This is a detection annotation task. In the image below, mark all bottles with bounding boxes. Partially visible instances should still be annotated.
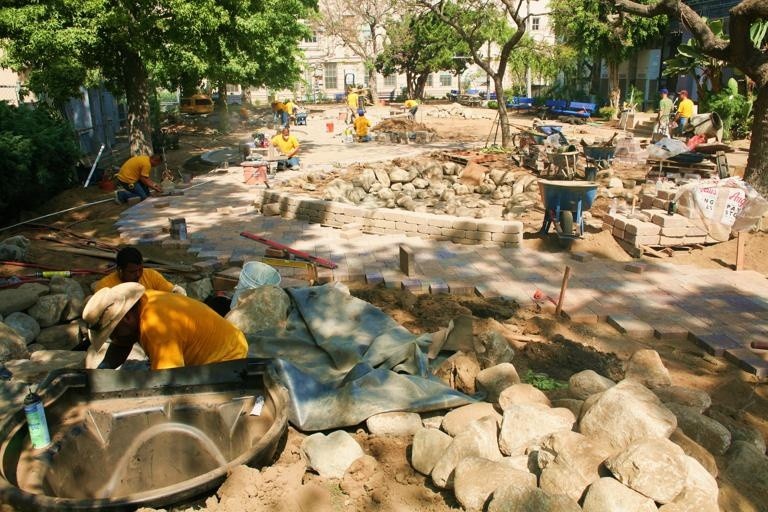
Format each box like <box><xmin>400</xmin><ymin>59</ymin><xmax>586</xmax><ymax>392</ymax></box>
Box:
<box><xmin>23</xmin><ymin>388</ymin><xmax>52</xmax><ymax>451</ymax></box>
<box><xmin>35</xmin><ymin>271</ymin><xmax>71</xmax><ymax>278</ymax></box>
<box><xmin>178</xmin><ymin>222</ymin><xmax>186</xmax><ymax>241</ymax></box>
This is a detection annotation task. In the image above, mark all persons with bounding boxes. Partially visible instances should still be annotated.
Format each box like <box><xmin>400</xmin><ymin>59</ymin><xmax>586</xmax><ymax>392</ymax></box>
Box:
<box><xmin>80</xmin><ymin>247</ymin><xmax>187</xmax><ymax>336</ymax></box>
<box><xmin>405</xmin><ymin>99</ymin><xmax>419</xmax><ymax>116</ymax></box>
<box><xmin>696</xmin><ymin>72</ymin><xmax>712</xmax><ymax>91</ymax></box>
<box><xmin>674</xmin><ymin>90</ymin><xmax>694</xmax><ymax>133</ymax></box>
<box><xmin>272</xmin><ymin>97</ymin><xmax>306</xmax><ymax>129</ymax></box>
<box><xmin>346</xmin><ymin>84</ymin><xmax>373</xmax><ymax>142</ymax></box>
<box><xmin>115</xmin><ymin>154</ymin><xmax>163</xmax><ymax>206</ymax></box>
<box><xmin>271</xmin><ymin>128</ymin><xmax>300</xmax><ymax>171</ymax></box>
<box><xmin>82</xmin><ymin>282</ymin><xmax>248</xmax><ymax>371</ymax></box>
<box><xmin>652</xmin><ymin>88</ymin><xmax>674</xmax><ymax>142</ymax></box>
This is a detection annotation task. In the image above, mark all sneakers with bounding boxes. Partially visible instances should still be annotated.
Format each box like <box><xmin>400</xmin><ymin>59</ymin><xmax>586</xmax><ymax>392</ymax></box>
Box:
<box><xmin>114</xmin><ymin>191</ymin><xmax>121</xmax><ymax>205</ymax></box>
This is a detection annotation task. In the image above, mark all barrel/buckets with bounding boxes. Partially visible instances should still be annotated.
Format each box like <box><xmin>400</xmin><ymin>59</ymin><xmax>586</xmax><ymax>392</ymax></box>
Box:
<box><xmin>339</xmin><ymin>112</ymin><xmax>348</xmax><ymax>122</ymax></box>
<box><xmin>326</xmin><ymin>121</ymin><xmax>333</xmax><ymax>132</ymax></box>
<box><xmin>230</xmin><ymin>258</ymin><xmax>280</xmax><ymax>310</ymax></box>
<box><xmin>240</xmin><ymin>161</ymin><xmax>268</xmax><ymax>184</ymax></box>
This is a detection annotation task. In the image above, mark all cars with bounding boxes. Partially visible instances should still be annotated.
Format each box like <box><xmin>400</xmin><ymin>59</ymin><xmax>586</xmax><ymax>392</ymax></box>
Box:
<box><xmin>179</xmin><ymin>92</ymin><xmax>214</xmax><ymax>114</ymax></box>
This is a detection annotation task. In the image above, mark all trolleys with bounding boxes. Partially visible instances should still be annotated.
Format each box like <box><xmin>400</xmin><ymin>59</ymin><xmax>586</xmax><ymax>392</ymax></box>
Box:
<box><xmin>535</xmin><ymin>139</ymin><xmax>616</xmax><ymax>248</ymax></box>
<box><xmin>295</xmin><ymin>113</ymin><xmax>308</xmax><ymax>125</ymax></box>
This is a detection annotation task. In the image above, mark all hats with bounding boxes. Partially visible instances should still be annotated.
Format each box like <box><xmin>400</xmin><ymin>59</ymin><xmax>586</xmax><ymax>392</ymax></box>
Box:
<box><xmin>677</xmin><ymin>90</ymin><xmax>688</xmax><ymax>95</ymax></box>
<box><xmin>658</xmin><ymin>88</ymin><xmax>668</xmax><ymax>94</ymax></box>
<box><xmin>82</xmin><ymin>282</ymin><xmax>145</xmax><ymax>353</ymax></box>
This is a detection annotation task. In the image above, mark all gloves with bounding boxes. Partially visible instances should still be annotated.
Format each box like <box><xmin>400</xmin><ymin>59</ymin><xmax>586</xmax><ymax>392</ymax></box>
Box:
<box><xmin>172</xmin><ymin>284</ymin><xmax>187</xmax><ymax>296</ymax></box>
